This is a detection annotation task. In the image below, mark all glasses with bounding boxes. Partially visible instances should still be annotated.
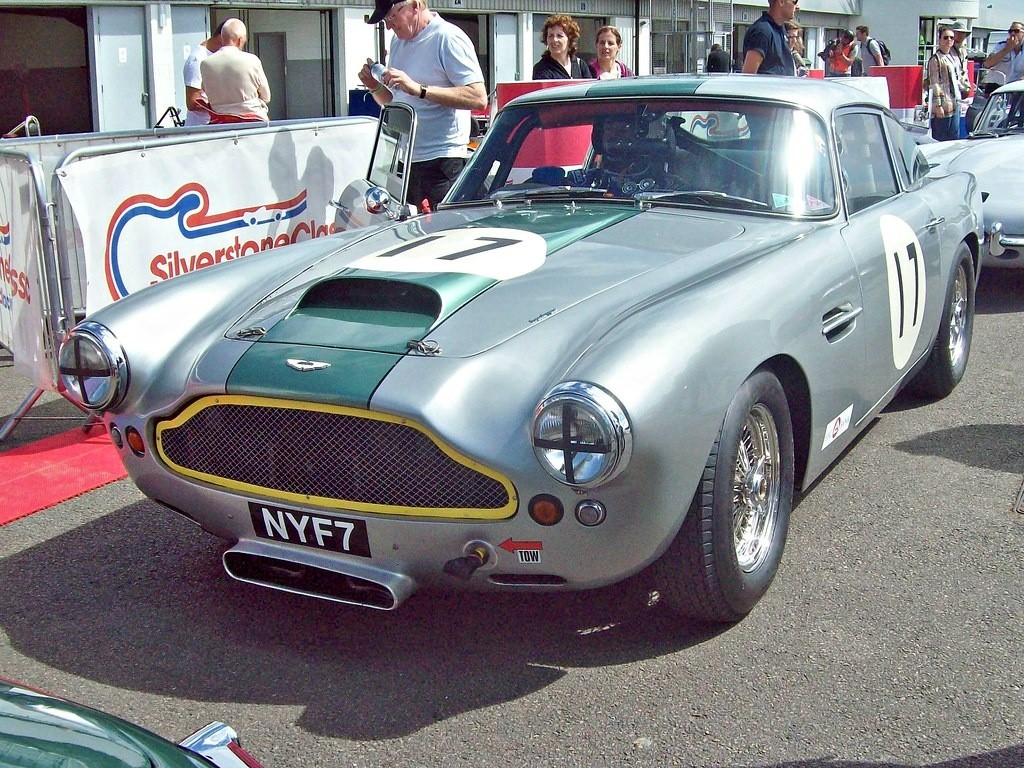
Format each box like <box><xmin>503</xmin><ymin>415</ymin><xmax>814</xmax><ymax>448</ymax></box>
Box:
<box><xmin>1008</xmin><ymin>28</ymin><xmax>1023</xmax><ymax>34</ymax></box>
<box><xmin>942</xmin><ymin>36</ymin><xmax>954</xmax><ymax>40</ymax></box>
<box><xmin>789</xmin><ymin>0</ymin><xmax>798</xmax><ymax>5</ymax></box>
<box><xmin>383</xmin><ymin>5</ymin><xmax>414</xmax><ymax>23</ymax></box>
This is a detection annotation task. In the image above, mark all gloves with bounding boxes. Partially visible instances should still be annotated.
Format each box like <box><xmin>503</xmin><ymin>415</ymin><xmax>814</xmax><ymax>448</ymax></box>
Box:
<box><xmin>532</xmin><ymin>166</ymin><xmax>565</xmax><ymax>186</ymax></box>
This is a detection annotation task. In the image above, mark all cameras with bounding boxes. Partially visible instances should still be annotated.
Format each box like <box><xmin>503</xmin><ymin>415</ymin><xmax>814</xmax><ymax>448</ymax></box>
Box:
<box><xmin>818</xmin><ymin>43</ymin><xmax>837</xmax><ymax>61</ymax></box>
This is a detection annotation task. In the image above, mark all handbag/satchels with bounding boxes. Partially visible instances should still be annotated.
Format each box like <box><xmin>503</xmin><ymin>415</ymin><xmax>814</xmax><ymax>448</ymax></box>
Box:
<box><xmin>931</xmin><ymin>93</ymin><xmax>953</xmax><ymax>113</ymax></box>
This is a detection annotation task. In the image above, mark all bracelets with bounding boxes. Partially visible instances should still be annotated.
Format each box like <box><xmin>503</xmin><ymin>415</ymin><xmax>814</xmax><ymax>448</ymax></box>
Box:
<box><xmin>936</xmin><ymin>104</ymin><xmax>942</xmax><ymax>107</ymax></box>
<box><xmin>367</xmin><ymin>84</ymin><xmax>384</xmax><ymax>95</ymax></box>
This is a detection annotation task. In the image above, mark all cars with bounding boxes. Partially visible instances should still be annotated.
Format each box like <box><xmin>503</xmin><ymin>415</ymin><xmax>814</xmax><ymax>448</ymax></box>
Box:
<box><xmin>1</xmin><ymin>676</ymin><xmax>264</xmax><ymax>768</ymax></box>
<box><xmin>57</xmin><ymin>74</ymin><xmax>984</xmax><ymax>618</ymax></box>
<box><xmin>917</xmin><ymin>79</ymin><xmax>1024</xmax><ymax>255</ymax></box>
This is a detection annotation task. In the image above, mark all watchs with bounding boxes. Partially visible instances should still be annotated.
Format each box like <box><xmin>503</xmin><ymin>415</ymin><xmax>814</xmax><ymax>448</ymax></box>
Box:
<box><xmin>419</xmin><ymin>82</ymin><xmax>429</xmax><ymax>99</ymax></box>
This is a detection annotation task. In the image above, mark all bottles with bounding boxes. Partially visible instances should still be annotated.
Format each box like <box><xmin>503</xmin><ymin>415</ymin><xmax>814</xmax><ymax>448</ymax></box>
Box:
<box><xmin>370</xmin><ymin>62</ymin><xmax>404</xmax><ymax>96</ymax></box>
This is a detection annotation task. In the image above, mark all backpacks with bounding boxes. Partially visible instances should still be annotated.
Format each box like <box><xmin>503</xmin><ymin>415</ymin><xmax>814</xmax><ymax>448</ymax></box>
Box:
<box><xmin>860</xmin><ymin>38</ymin><xmax>891</xmax><ymax>66</ymax></box>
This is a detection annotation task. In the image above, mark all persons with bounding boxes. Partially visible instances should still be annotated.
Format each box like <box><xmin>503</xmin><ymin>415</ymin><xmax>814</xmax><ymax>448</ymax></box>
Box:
<box><xmin>985</xmin><ymin>22</ymin><xmax>1024</xmax><ymax>85</ymax></box>
<box><xmin>588</xmin><ymin>26</ymin><xmax>636</xmax><ymax>79</ymax></box>
<box><xmin>856</xmin><ymin>25</ymin><xmax>885</xmax><ymax>77</ymax></box>
<box><xmin>358</xmin><ymin>0</ymin><xmax>489</xmax><ymax>210</ymax></box>
<box><xmin>926</xmin><ymin>21</ymin><xmax>988</xmax><ymax>141</ymax></box>
<box><xmin>740</xmin><ymin>0</ymin><xmax>810</xmax><ymax>77</ymax></box>
<box><xmin>200</xmin><ymin>18</ymin><xmax>271</xmax><ymax>124</ymax></box>
<box><xmin>566</xmin><ymin>113</ymin><xmax>684</xmax><ymax>191</ymax></box>
<box><xmin>817</xmin><ymin>29</ymin><xmax>859</xmax><ymax>77</ymax></box>
<box><xmin>703</xmin><ymin>44</ymin><xmax>732</xmax><ymax>75</ymax></box>
<box><xmin>532</xmin><ymin>14</ymin><xmax>593</xmax><ymax>80</ymax></box>
<box><xmin>183</xmin><ymin>17</ymin><xmax>227</xmax><ymax>127</ymax></box>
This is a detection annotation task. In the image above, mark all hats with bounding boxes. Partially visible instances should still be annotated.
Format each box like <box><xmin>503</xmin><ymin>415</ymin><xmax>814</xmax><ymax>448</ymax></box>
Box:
<box><xmin>951</xmin><ymin>21</ymin><xmax>971</xmax><ymax>37</ymax></box>
<box><xmin>366</xmin><ymin>0</ymin><xmax>409</xmax><ymax>24</ymax></box>
